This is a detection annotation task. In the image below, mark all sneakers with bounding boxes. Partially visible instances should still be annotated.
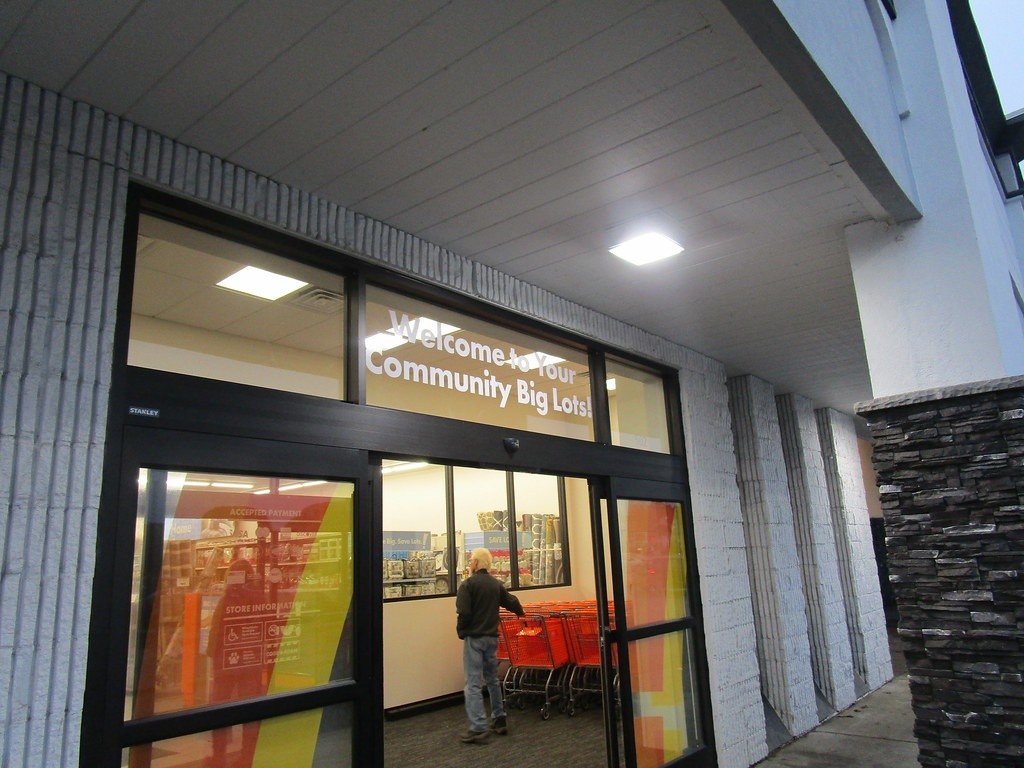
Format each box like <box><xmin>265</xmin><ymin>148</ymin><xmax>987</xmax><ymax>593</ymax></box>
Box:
<box><xmin>489</xmin><ymin>716</ymin><xmax>508</xmax><ymax>734</ymax></box>
<box><xmin>460</xmin><ymin>725</ymin><xmax>489</xmax><ymax>742</ymax></box>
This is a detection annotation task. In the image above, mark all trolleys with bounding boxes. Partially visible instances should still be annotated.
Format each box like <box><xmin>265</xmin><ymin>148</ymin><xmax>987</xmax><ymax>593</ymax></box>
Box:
<box><xmin>496</xmin><ymin>599</ymin><xmax>618</xmax><ymax>721</ymax></box>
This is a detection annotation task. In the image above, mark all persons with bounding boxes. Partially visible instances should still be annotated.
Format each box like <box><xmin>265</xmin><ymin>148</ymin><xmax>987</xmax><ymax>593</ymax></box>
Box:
<box><xmin>456</xmin><ymin>548</ymin><xmax>526</xmax><ymax>740</ymax></box>
<box><xmin>205</xmin><ymin>559</ymin><xmax>268</xmax><ymax>768</ymax></box>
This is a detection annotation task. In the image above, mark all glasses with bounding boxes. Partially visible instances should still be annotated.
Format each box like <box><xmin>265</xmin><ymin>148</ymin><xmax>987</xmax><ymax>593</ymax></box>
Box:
<box><xmin>468</xmin><ymin>559</ymin><xmax>476</xmax><ymax>564</ymax></box>
